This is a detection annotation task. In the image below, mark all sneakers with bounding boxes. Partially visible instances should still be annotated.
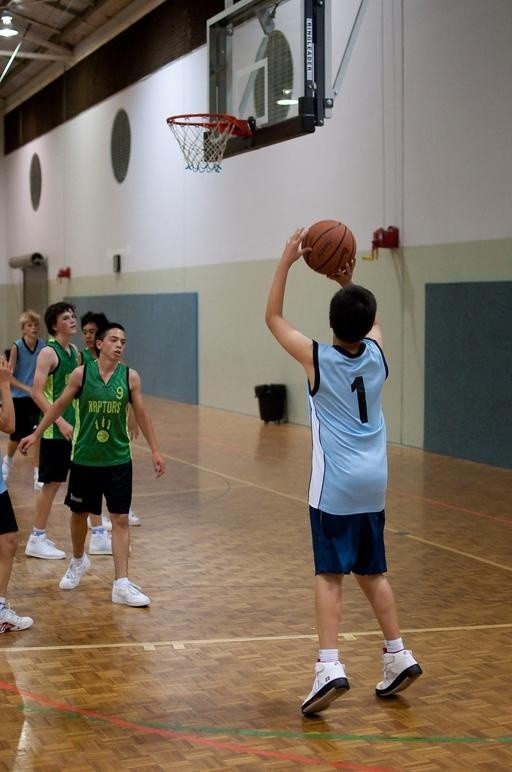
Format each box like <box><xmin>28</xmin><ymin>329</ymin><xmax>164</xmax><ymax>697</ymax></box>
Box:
<box><xmin>0</xmin><ymin>608</ymin><xmax>33</xmax><ymax>633</ymax></box>
<box><xmin>111</xmin><ymin>578</ymin><xmax>151</xmax><ymax>607</ymax></box>
<box><xmin>89</xmin><ymin>531</ymin><xmax>131</xmax><ymax>555</ymax></box>
<box><xmin>59</xmin><ymin>554</ymin><xmax>92</xmax><ymax>590</ymax></box>
<box><xmin>301</xmin><ymin>661</ymin><xmax>350</xmax><ymax>716</ymax></box>
<box><xmin>87</xmin><ymin>509</ymin><xmax>140</xmax><ymax>530</ymax></box>
<box><xmin>25</xmin><ymin>531</ymin><xmax>64</xmax><ymax>560</ymax></box>
<box><xmin>376</xmin><ymin>650</ymin><xmax>423</xmax><ymax>698</ymax></box>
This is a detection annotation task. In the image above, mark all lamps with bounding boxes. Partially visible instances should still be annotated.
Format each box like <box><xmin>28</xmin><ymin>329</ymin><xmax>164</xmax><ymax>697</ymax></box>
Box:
<box><xmin>0</xmin><ymin>8</ymin><xmax>19</xmax><ymax>37</ymax></box>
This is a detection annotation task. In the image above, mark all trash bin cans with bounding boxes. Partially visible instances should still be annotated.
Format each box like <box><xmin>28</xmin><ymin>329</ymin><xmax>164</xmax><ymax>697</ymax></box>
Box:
<box><xmin>255</xmin><ymin>384</ymin><xmax>286</xmax><ymax>424</ymax></box>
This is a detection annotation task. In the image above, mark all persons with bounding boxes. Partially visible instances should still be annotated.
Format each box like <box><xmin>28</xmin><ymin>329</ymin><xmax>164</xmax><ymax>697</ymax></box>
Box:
<box><xmin>265</xmin><ymin>225</ymin><xmax>422</xmax><ymax>715</ymax></box>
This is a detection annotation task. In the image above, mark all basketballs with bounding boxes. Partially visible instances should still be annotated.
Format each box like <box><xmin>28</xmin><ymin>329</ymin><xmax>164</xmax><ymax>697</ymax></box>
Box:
<box><xmin>301</xmin><ymin>220</ymin><xmax>356</xmax><ymax>275</ymax></box>
